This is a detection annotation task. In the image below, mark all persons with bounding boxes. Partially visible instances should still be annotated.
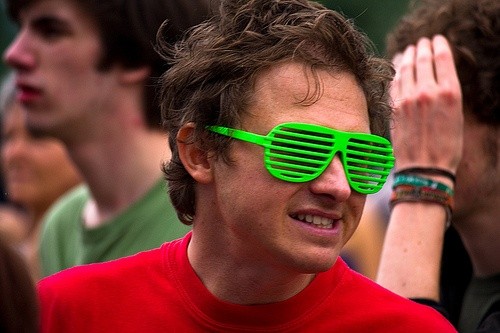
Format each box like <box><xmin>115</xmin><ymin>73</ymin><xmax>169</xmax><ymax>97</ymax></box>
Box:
<box><xmin>0</xmin><ymin>70</ymin><xmax>84</xmax><ymax>260</ymax></box>
<box><xmin>375</xmin><ymin>0</ymin><xmax>500</xmax><ymax>333</ymax></box>
<box><xmin>34</xmin><ymin>0</ymin><xmax>459</xmax><ymax>333</ymax></box>
<box><xmin>4</xmin><ymin>0</ymin><xmax>210</xmax><ymax>280</ymax></box>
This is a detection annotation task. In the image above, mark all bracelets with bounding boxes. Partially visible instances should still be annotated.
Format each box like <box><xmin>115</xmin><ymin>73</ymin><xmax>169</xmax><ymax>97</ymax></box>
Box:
<box><xmin>388</xmin><ymin>166</ymin><xmax>457</xmax><ymax>229</ymax></box>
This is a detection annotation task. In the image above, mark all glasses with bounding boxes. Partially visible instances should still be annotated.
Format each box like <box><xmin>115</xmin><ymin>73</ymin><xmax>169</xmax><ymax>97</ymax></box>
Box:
<box><xmin>209</xmin><ymin>123</ymin><xmax>395</xmax><ymax>195</ymax></box>
